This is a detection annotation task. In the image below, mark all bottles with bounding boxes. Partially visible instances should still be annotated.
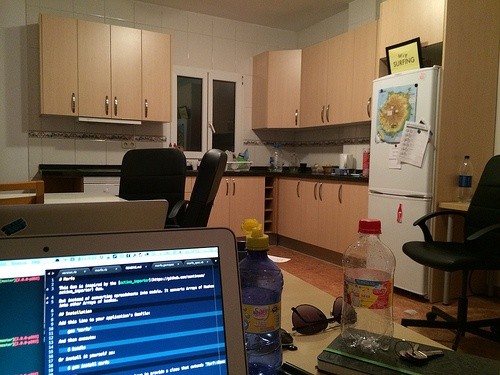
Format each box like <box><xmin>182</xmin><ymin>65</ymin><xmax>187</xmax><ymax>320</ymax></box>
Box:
<box><xmin>270</xmin><ymin>144</ymin><xmax>282</xmax><ymax>172</ymax></box>
<box><xmin>239</xmin><ymin>219</ymin><xmax>284</xmax><ymax>375</ymax></box>
<box><xmin>457</xmin><ymin>155</ymin><xmax>473</xmax><ymax>206</ymax></box>
<box><xmin>169</xmin><ymin>142</ymin><xmax>176</xmax><ymax>147</ymax></box>
<box><xmin>340</xmin><ymin>218</ymin><xmax>396</xmax><ymax>354</ymax></box>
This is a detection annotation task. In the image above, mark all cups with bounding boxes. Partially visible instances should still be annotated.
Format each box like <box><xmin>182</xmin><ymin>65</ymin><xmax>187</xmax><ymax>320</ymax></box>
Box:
<box><xmin>192</xmin><ymin>160</ymin><xmax>198</xmax><ymax>170</ymax></box>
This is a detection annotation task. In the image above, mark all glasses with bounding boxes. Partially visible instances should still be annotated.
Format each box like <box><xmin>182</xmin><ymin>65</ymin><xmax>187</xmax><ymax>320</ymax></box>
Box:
<box><xmin>290</xmin><ymin>296</ymin><xmax>358</xmax><ymax>336</ymax></box>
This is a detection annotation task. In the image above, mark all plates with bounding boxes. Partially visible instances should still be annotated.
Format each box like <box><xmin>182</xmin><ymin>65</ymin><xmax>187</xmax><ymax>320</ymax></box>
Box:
<box><xmin>351</xmin><ymin>174</ymin><xmax>360</xmax><ymax>177</ymax></box>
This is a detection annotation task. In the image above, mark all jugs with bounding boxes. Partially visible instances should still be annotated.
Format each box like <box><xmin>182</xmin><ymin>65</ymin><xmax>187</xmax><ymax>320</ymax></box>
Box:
<box><xmin>346</xmin><ymin>154</ymin><xmax>356</xmax><ymax>169</ymax></box>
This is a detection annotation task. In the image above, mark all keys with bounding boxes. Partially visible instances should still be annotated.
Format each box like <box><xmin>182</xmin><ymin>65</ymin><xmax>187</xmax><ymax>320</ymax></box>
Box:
<box><xmin>399</xmin><ymin>344</ymin><xmax>444</xmax><ymax>362</ymax></box>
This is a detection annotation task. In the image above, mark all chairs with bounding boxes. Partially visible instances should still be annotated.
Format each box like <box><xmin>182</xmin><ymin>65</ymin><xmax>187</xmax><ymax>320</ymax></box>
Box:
<box><xmin>400</xmin><ymin>155</ymin><xmax>500</xmax><ymax>350</ymax></box>
<box><xmin>37</xmin><ymin>166</ymin><xmax>91</xmax><ymax>194</ymax></box>
<box><xmin>114</xmin><ymin>148</ymin><xmax>236</xmax><ymax>230</ymax></box>
<box><xmin>0</xmin><ymin>180</ymin><xmax>48</xmax><ymax>209</ymax></box>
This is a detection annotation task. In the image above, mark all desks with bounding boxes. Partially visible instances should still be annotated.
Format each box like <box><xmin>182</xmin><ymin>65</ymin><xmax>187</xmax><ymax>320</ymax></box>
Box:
<box><xmin>437</xmin><ymin>187</ymin><xmax>474</xmax><ymax>247</ymax></box>
<box><xmin>246</xmin><ymin>256</ymin><xmax>453</xmax><ymax>375</ymax></box>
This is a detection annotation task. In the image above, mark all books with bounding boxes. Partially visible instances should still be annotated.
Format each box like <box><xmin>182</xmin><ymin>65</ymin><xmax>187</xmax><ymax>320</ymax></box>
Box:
<box><xmin>317</xmin><ymin>327</ymin><xmax>500</xmax><ymax>375</ymax></box>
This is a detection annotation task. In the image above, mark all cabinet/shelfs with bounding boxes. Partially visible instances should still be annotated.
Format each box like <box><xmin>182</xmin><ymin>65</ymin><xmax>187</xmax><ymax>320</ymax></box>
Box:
<box><xmin>248</xmin><ymin>17</ymin><xmax>379</xmax><ymax>134</ymax></box>
<box><xmin>277</xmin><ymin>169</ymin><xmax>371</xmax><ymax>264</ymax></box>
<box><xmin>36</xmin><ymin>10</ymin><xmax>174</xmax><ymax>130</ymax></box>
<box><xmin>182</xmin><ymin>169</ymin><xmax>273</xmax><ymax>239</ymax></box>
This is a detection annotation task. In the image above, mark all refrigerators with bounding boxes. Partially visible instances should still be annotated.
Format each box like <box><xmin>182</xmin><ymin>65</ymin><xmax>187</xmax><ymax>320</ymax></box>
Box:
<box><xmin>368</xmin><ymin>64</ymin><xmax>442</xmax><ymax>300</ymax></box>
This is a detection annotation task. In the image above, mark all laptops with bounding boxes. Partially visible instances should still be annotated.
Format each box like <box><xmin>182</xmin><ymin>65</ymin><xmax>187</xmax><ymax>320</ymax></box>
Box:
<box><xmin>0</xmin><ymin>227</ymin><xmax>250</xmax><ymax>375</ymax></box>
<box><xmin>0</xmin><ymin>199</ymin><xmax>166</xmax><ymax>241</ymax></box>
